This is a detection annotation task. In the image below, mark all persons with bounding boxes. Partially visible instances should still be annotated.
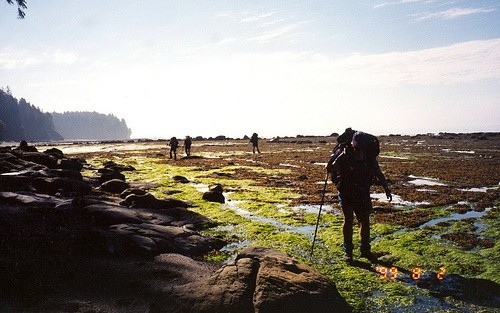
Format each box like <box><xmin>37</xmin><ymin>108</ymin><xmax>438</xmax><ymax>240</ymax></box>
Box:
<box><xmin>169</xmin><ymin>137</ymin><xmax>178</xmax><ymax>160</ymax></box>
<box><xmin>251</xmin><ymin>133</ymin><xmax>261</xmax><ymax>154</ymax></box>
<box><xmin>184</xmin><ymin>136</ymin><xmax>192</xmax><ymax>157</ymax></box>
<box><xmin>326</xmin><ymin>128</ymin><xmax>392</xmax><ymax>262</ymax></box>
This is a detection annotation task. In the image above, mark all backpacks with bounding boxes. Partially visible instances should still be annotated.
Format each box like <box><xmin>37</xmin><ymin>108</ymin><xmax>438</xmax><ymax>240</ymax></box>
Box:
<box><xmin>325</xmin><ymin>131</ymin><xmax>379</xmax><ymax>191</ymax></box>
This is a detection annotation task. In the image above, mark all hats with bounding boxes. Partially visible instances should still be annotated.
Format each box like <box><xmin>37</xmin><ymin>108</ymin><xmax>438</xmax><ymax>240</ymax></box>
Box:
<box><xmin>337</xmin><ymin>128</ymin><xmax>358</xmax><ymax>142</ymax></box>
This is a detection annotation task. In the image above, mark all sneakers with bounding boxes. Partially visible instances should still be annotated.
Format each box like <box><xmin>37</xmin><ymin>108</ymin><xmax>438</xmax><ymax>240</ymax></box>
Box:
<box><xmin>360</xmin><ymin>251</ymin><xmax>372</xmax><ymax>258</ymax></box>
<box><xmin>343</xmin><ymin>248</ymin><xmax>353</xmax><ymax>262</ymax></box>
